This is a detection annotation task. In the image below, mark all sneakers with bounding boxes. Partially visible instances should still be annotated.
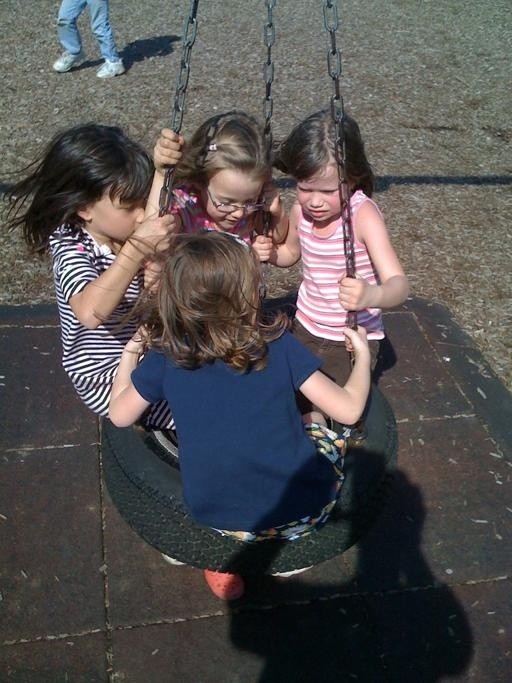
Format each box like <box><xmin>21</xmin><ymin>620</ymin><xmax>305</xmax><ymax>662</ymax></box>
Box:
<box><xmin>201</xmin><ymin>567</ymin><xmax>245</xmax><ymax>602</ymax></box>
<box><xmin>53</xmin><ymin>49</ymin><xmax>88</xmax><ymax>72</ymax></box>
<box><xmin>96</xmin><ymin>57</ymin><xmax>126</xmax><ymax>77</ymax></box>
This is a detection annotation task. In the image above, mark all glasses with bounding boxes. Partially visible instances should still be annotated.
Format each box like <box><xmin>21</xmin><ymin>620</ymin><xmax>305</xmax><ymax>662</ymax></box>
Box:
<box><xmin>205</xmin><ymin>187</ymin><xmax>267</xmax><ymax>213</ymax></box>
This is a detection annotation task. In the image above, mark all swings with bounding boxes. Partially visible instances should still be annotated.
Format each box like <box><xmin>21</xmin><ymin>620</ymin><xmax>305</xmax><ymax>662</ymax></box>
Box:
<box><xmin>99</xmin><ymin>1</ymin><xmax>399</xmax><ymax>575</ymax></box>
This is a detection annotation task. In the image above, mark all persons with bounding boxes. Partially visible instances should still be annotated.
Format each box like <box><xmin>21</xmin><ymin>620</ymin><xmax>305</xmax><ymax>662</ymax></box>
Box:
<box><xmin>109</xmin><ymin>227</ymin><xmax>372</xmax><ymax>601</ymax></box>
<box><xmin>52</xmin><ymin>0</ymin><xmax>126</xmax><ymax>78</ymax></box>
<box><xmin>142</xmin><ymin>109</ymin><xmax>289</xmax><ymax>256</ymax></box>
<box><xmin>250</xmin><ymin>104</ymin><xmax>410</xmax><ymax>423</ymax></box>
<box><xmin>0</xmin><ymin>123</ymin><xmax>177</xmax><ymax>431</ymax></box>
